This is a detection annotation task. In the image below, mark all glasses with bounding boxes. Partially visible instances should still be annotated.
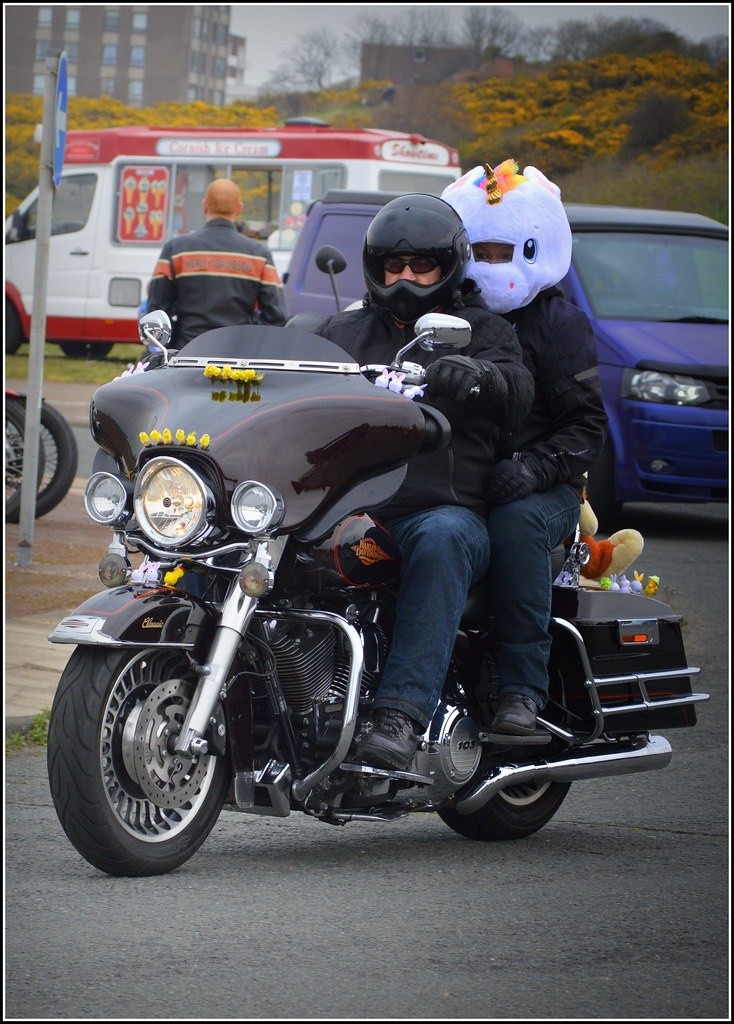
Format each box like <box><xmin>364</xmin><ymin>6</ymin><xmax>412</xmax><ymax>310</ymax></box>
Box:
<box><xmin>380</xmin><ymin>256</ymin><xmax>440</xmax><ymax>275</ymax></box>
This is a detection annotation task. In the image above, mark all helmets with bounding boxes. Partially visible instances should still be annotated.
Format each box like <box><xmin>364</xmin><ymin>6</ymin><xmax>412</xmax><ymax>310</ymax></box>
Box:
<box><xmin>362</xmin><ymin>192</ymin><xmax>471</xmax><ymax>327</ymax></box>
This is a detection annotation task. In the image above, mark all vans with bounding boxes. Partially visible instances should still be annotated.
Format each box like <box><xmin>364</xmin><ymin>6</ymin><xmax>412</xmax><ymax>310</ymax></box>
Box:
<box><xmin>5</xmin><ymin>118</ymin><xmax>462</xmax><ymax>358</ymax></box>
<box><xmin>280</xmin><ymin>185</ymin><xmax>728</xmax><ymax>527</ymax></box>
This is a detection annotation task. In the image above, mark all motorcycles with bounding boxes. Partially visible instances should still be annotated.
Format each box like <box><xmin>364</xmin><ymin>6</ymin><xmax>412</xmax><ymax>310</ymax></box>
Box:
<box><xmin>47</xmin><ymin>244</ymin><xmax>711</xmax><ymax>877</ymax></box>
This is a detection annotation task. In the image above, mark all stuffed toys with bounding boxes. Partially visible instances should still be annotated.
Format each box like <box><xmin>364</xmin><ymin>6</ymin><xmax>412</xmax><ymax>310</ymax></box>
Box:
<box><xmin>568</xmin><ymin>494</ymin><xmax>662</xmax><ymax>598</ymax></box>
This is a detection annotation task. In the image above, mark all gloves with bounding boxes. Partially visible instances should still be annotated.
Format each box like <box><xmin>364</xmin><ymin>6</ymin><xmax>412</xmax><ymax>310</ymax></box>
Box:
<box><xmin>426</xmin><ymin>354</ymin><xmax>491</xmax><ymax>405</ymax></box>
<box><xmin>484</xmin><ymin>453</ymin><xmax>549</xmax><ymax>508</ymax></box>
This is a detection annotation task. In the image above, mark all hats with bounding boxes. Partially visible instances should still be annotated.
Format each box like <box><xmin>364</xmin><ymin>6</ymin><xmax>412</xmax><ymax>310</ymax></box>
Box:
<box><xmin>440</xmin><ymin>159</ymin><xmax>572</xmax><ymax>314</ymax></box>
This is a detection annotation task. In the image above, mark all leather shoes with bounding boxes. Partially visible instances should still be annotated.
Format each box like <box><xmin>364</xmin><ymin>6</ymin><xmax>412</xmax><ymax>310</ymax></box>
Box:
<box><xmin>354</xmin><ymin>708</ymin><xmax>419</xmax><ymax>770</ymax></box>
<box><xmin>491</xmin><ymin>693</ymin><xmax>537</xmax><ymax>736</ymax></box>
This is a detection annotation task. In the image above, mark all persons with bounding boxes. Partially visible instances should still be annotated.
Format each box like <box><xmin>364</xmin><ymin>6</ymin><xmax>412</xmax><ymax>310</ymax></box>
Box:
<box><xmin>437</xmin><ymin>161</ymin><xmax>611</xmax><ymax>734</ymax></box>
<box><xmin>313</xmin><ymin>192</ymin><xmax>536</xmax><ymax>774</ymax></box>
<box><xmin>146</xmin><ymin>178</ymin><xmax>288</xmax><ymax>348</ymax></box>
